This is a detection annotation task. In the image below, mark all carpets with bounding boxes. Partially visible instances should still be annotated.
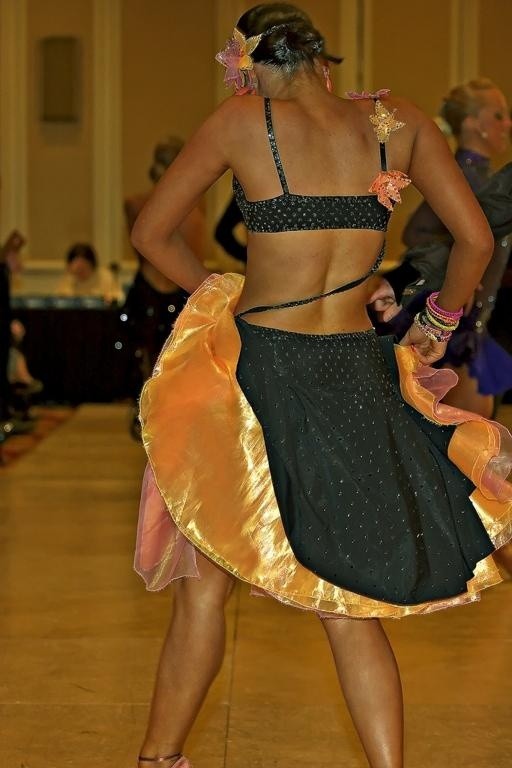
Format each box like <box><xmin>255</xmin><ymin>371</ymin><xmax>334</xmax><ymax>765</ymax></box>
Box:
<box><xmin>0</xmin><ymin>406</ymin><xmax>79</xmax><ymax>473</ymax></box>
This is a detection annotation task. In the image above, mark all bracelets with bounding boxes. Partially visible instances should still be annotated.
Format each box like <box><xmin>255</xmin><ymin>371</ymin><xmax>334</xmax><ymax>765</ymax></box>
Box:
<box><xmin>413</xmin><ymin>289</ymin><xmax>464</xmax><ymax>344</ymax></box>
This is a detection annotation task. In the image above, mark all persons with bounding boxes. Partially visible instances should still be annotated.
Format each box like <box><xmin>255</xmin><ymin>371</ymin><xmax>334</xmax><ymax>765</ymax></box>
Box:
<box><xmin>123</xmin><ymin>134</ymin><xmax>206</xmax><ymax>442</ymax></box>
<box><xmin>399</xmin><ymin>75</ymin><xmax>511</xmax><ymax>421</ymax></box>
<box><xmin>128</xmin><ymin>3</ymin><xmax>511</xmax><ymax>767</ymax></box>
<box><xmin>55</xmin><ymin>242</ymin><xmax>122</xmax><ymax>301</ymax></box>
<box><xmin>366</xmin><ymin>163</ymin><xmax>511</xmax><ymax>358</ymax></box>
